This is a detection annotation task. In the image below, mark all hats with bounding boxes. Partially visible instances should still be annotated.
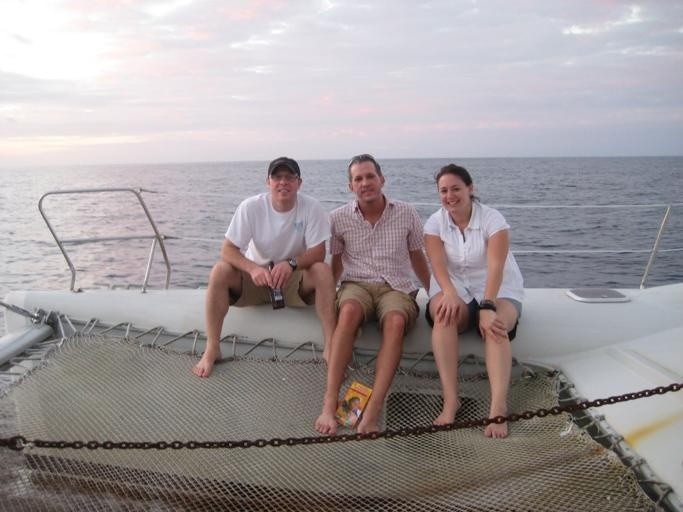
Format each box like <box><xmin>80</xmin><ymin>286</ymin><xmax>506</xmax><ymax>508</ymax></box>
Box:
<box><xmin>268</xmin><ymin>157</ymin><xmax>300</xmax><ymax>177</ymax></box>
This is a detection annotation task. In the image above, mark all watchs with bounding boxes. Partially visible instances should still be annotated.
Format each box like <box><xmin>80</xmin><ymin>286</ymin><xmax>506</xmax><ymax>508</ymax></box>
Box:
<box><xmin>285</xmin><ymin>258</ymin><xmax>297</xmax><ymax>271</ymax></box>
<box><xmin>479</xmin><ymin>299</ymin><xmax>496</xmax><ymax>312</ymax></box>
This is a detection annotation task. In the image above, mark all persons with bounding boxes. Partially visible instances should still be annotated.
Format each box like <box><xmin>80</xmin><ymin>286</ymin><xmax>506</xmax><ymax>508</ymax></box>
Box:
<box><xmin>422</xmin><ymin>163</ymin><xmax>526</xmax><ymax>439</ymax></box>
<box><xmin>191</xmin><ymin>156</ymin><xmax>350</xmax><ymax>381</ymax></box>
<box><xmin>314</xmin><ymin>153</ymin><xmax>429</xmax><ymax>441</ymax></box>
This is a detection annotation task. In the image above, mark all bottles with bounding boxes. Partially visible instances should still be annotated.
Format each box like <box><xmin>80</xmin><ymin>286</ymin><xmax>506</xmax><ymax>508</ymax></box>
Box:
<box><xmin>267</xmin><ymin>260</ymin><xmax>285</xmax><ymax>310</ymax></box>
